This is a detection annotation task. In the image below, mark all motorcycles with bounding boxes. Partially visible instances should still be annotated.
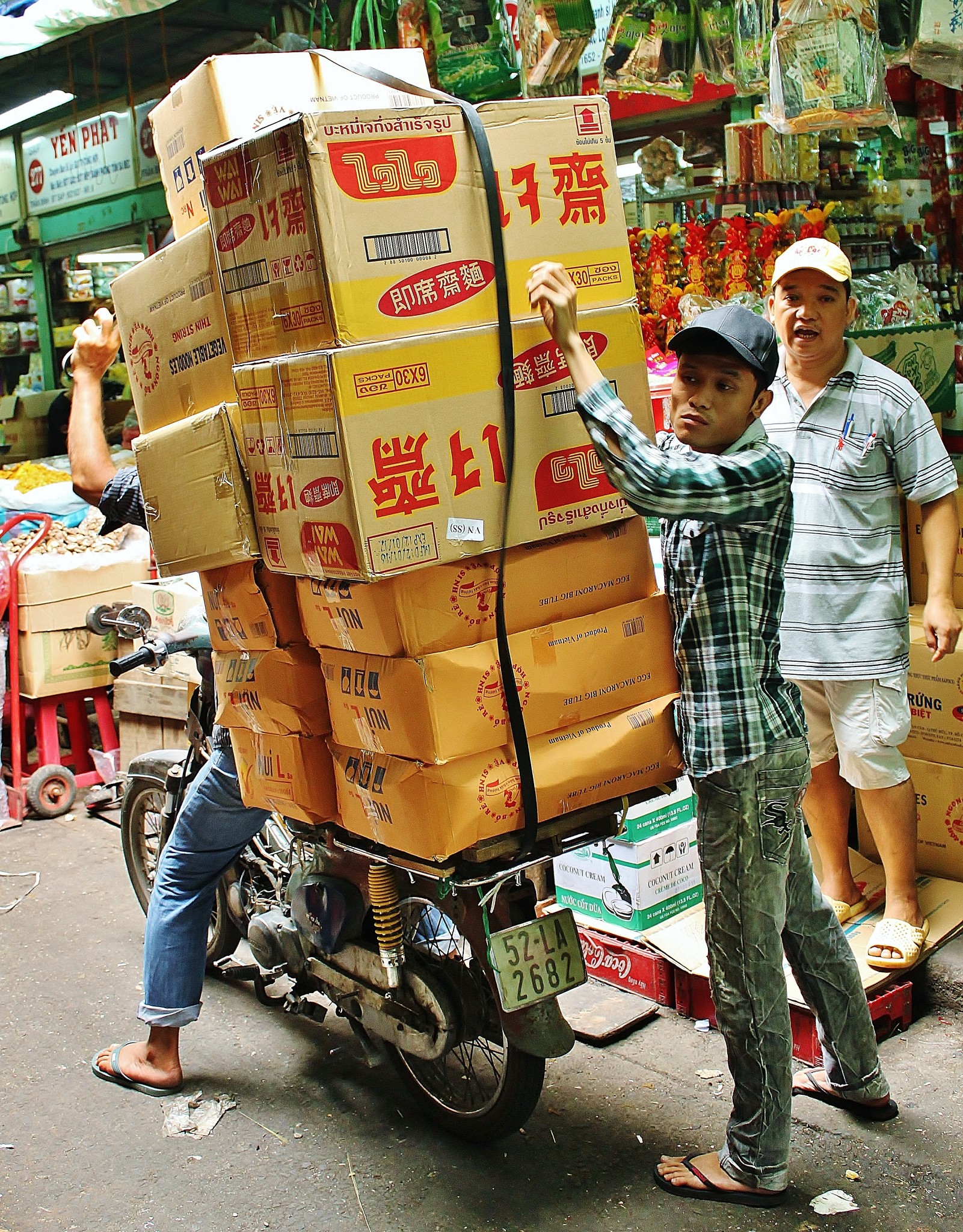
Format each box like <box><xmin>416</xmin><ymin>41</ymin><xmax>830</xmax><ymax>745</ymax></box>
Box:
<box><xmin>108</xmin><ymin>605</ymin><xmax>590</xmax><ymax>1145</ymax></box>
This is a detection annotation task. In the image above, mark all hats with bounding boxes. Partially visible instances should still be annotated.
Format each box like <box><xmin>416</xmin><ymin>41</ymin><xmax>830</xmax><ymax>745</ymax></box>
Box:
<box><xmin>668</xmin><ymin>306</ymin><xmax>780</xmax><ymax>379</ymax></box>
<box><xmin>772</xmin><ymin>237</ymin><xmax>853</xmax><ymax>288</ymax></box>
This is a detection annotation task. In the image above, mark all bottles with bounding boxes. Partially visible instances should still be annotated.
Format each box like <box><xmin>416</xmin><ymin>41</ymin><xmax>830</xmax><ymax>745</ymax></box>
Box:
<box><xmin>715</xmin><ymin>151</ymin><xmax>963</xmax><ymax>322</ymax></box>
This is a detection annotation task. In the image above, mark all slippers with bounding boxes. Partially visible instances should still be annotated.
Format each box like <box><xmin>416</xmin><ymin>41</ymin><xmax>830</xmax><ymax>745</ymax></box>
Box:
<box><xmin>867</xmin><ymin>919</ymin><xmax>930</xmax><ymax>969</ymax></box>
<box><xmin>821</xmin><ymin>895</ymin><xmax>868</xmax><ymax>923</ymax></box>
<box><xmin>655</xmin><ymin>1151</ymin><xmax>787</xmax><ymax>1207</ymax></box>
<box><xmin>792</xmin><ymin>1068</ymin><xmax>900</xmax><ymax>1123</ymax></box>
<box><xmin>91</xmin><ymin>1041</ymin><xmax>183</xmax><ymax>1098</ymax></box>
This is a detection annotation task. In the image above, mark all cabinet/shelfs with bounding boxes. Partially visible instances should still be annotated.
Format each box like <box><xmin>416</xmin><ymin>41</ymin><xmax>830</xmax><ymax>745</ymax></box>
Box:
<box><xmin>50</xmin><ymin>252</ymin><xmax>145</xmax><ymax>401</ymax></box>
<box><xmin>635</xmin><ymin>172</ymin><xmax>716</xmax><ymax>248</ymax></box>
<box><xmin>815</xmin><ymin>139</ymin><xmax>894</xmax><ymax>277</ymax></box>
<box><xmin>0</xmin><ymin>271</ymin><xmax>41</xmax><ymax>397</ymax></box>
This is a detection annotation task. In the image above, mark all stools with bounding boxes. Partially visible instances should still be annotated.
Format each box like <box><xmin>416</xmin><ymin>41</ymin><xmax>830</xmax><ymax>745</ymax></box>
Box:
<box><xmin>0</xmin><ymin>687</ymin><xmax>120</xmax><ymax>819</ymax></box>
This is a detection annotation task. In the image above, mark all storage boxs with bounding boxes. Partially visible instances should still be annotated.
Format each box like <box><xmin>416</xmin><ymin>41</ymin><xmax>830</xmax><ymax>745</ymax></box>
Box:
<box><xmin>0</xmin><ymin>46</ymin><xmax>963</xmax><ymax>1069</ymax></box>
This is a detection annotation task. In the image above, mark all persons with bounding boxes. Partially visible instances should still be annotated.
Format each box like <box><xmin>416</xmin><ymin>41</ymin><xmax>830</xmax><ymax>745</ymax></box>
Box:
<box><xmin>63</xmin><ymin>309</ymin><xmax>468</xmax><ymax>1097</ymax></box>
<box><xmin>528</xmin><ymin>261</ymin><xmax>898</xmax><ymax>1209</ymax></box>
<box><xmin>751</xmin><ymin>236</ymin><xmax>962</xmax><ymax>972</ymax></box>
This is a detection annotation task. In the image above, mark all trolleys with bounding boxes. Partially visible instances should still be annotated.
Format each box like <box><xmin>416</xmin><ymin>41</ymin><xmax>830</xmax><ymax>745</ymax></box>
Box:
<box><xmin>0</xmin><ymin>509</ymin><xmax>120</xmax><ymax>835</ymax></box>
